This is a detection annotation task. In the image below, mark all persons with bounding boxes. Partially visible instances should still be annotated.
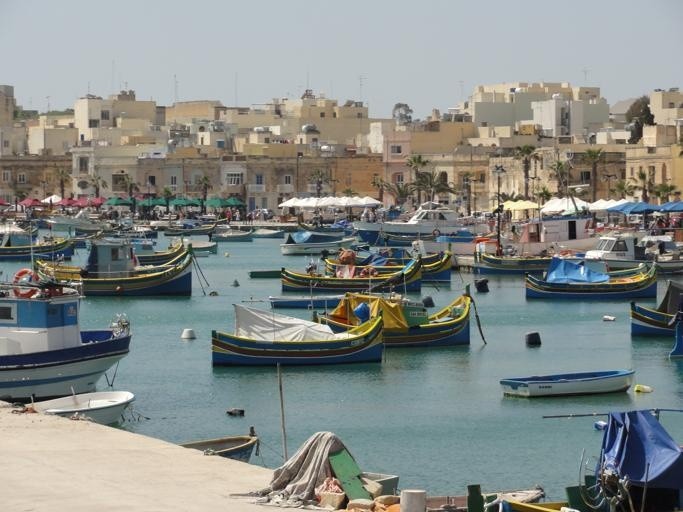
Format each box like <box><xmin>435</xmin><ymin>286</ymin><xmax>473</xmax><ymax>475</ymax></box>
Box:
<box><xmin>224</xmin><ymin>207</ymin><xmax>232</xmax><ymax>225</ymax></box>
<box><xmin>245</xmin><ymin>205</ymin><xmax>278</xmax><ymax>224</ymax></box>
<box><xmin>488</xmin><ymin>216</ymin><xmax>495</xmax><ymax>232</ymax></box>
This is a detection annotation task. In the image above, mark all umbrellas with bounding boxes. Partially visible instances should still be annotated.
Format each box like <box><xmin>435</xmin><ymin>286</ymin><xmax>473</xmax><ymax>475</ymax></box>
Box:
<box><xmin>495</xmin><ymin>196</ymin><xmax>683</xmax><ymax>229</ymax></box>
<box><xmin>276</xmin><ymin>195</ymin><xmax>383</xmax><ymax>222</ymax></box>
<box><xmin>0</xmin><ymin>197</ymin><xmax>11</xmax><ymax>206</ymax></box>
<box><xmin>14</xmin><ymin>191</ymin><xmax>247</xmax><ymax>220</ymax></box>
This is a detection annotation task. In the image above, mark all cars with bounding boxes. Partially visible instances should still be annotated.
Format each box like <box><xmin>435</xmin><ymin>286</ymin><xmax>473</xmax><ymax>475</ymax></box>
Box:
<box><xmin>3</xmin><ymin>204</ymin><xmax>108</xmax><ymax>215</ymax></box>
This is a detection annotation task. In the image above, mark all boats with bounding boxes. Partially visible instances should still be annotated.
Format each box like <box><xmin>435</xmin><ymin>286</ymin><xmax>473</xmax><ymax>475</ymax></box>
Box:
<box><xmin>499</xmin><ymin>369</ymin><xmax>635</xmax><ymax>399</ymax></box>
<box><xmin>629</xmin><ymin>279</ymin><xmax>683</xmax><ymax>338</ymax></box>
<box><xmin>269</xmin><ymin>294</ymin><xmax>344</xmax><ymax>309</ymax></box>
<box><xmin>210</xmin><ymin>302</ymin><xmax>384</xmax><ymax>367</ymax></box>
<box><xmin>668</xmin><ymin>310</ymin><xmax>683</xmax><ymax>357</ymax></box>
<box><xmin>320</xmin><ymin>241</ymin><xmax>454</xmax><ymax>283</ymax></box>
<box><xmin>363</xmin><ymin>471</ymin><xmax>569</xmax><ymax>512</ymax></box>
<box><xmin>311</xmin><ymin>283</ymin><xmax>472</xmax><ymax>348</ymax></box>
<box><xmin>280</xmin><ymin>253</ymin><xmax>423</xmax><ymax>293</ymax></box>
<box><xmin>163</xmin><ymin>166</ymin><xmax>682</xmax><ymax>276</ymax></box>
<box><xmin>177</xmin><ymin>426</ymin><xmax>258</xmax><ymax>463</ymax></box>
<box><xmin>524</xmin><ymin>256</ymin><xmax>658</xmax><ymax>301</ymax></box>
<box><xmin>0</xmin><ymin>216</ymin><xmax>192</xmax><ymax>425</ymax></box>
